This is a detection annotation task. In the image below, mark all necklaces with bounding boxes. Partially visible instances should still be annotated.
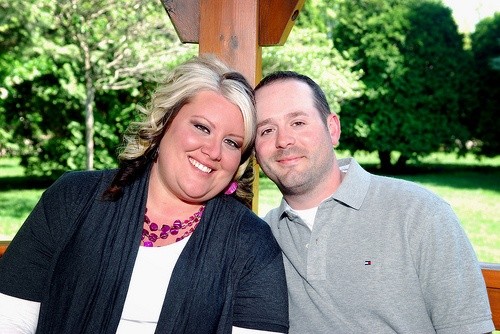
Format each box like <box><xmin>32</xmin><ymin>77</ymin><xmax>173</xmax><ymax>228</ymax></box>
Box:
<box><xmin>140</xmin><ymin>201</ymin><xmax>208</xmax><ymax>249</ymax></box>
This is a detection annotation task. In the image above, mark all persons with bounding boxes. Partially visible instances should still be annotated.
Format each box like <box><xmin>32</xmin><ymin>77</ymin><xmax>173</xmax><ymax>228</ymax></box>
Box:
<box><xmin>252</xmin><ymin>69</ymin><xmax>496</xmax><ymax>334</ymax></box>
<box><xmin>0</xmin><ymin>53</ymin><xmax>290</xmax><ymax>334</ymax></box>
